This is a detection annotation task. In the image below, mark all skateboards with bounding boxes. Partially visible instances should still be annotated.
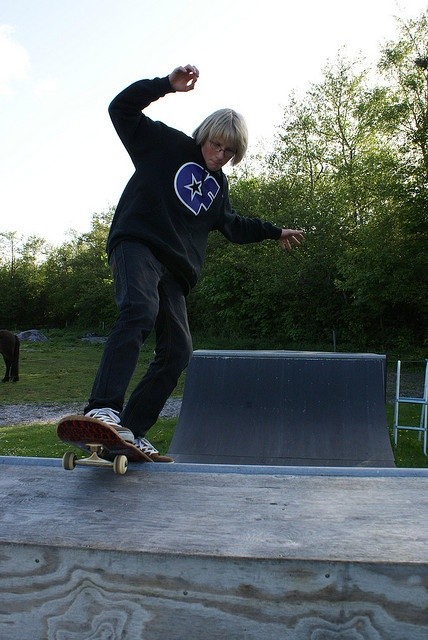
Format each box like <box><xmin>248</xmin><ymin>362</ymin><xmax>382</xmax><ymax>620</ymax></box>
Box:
<box><xmin>57</xmin><ymin>415</ymin><xmax>154</xmax><ymax>475</ymax></box>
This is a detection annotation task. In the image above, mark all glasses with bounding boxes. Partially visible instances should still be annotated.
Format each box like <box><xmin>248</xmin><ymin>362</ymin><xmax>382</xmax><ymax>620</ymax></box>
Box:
<box><xmin>209</xmin><ymin>140</ymin><xmax>234</xmax><ymax>159</ymax></box>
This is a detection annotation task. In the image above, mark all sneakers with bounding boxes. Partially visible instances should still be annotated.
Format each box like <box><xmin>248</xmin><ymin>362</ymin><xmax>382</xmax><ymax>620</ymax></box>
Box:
<box><xmin>133</xmin><ymin>437</ymin><xmax>173</xmax><ymax>463</ymax></box>
<box><xmin>84</xmin><ymin>408</ymin><xmax>134</xmax><ymax>443</ymax></box>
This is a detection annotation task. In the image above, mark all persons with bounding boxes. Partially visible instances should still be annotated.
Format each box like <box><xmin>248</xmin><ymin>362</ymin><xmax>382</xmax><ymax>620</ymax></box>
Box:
<box><xmin>83</xmin><ymin>64</ymin><xmax>304</xmax><ymax>464</ymax></box>
